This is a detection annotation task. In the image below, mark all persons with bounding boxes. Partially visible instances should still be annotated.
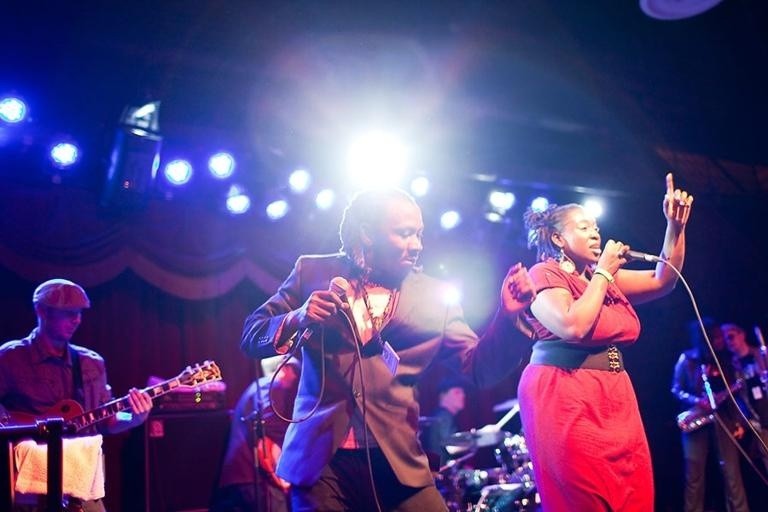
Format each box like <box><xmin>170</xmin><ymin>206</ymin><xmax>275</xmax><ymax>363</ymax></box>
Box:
<box><xmin>0</xmin><ymin>277</ymin><xmax>153</xmax><ymax>512</ymax></box>
<box><xmin>672</xmin><ymin>317</ymin><xmax>750</xmax><ymax>511</ymax></box>
<box><xmin>220</xmin><ymin>356</ymin><xmax>302</xmax><ymax>512</ymax></box>
<box><xmin>418</xmin><ymin>378</ymin><xmax>467</xmax><ymax>494</ymax></box>
<box><xmin>518</xmin><ymin>173</ymin><xmax>692</xmax><ymax>512</ymax></box>
<box><xmin>720</xmin><ymin>321</ymin><xmax>768</xmax><ymax>470</ymax></box>
<box><xmin>239</xmin><ymin>185</ymin><xmax>538</xmax><ymax>512</ymax></box>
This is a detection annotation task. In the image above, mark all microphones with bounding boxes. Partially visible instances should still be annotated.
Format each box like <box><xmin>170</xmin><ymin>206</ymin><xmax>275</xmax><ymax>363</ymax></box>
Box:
<box><xmin>621</xmin><ymin>246</ymin><xmax>653</xmax><ymax>264</ymax></box>
<box><xmin>294</xmin><ymin>277</ymin><xmax>350</xmax><ymax>343</ymax></box>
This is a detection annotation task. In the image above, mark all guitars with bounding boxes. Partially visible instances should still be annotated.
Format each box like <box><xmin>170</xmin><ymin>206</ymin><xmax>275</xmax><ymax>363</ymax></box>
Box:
<box><xmin>1</xmin><ymin>362</ymin><xmax>223</xmax><ymax>445</ymax></box>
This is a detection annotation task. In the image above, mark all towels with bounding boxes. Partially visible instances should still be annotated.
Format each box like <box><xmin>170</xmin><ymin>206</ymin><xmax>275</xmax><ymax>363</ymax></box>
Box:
<box><xmin>13</xmin><ymin>431</ymin><xmax>108</xmax><ymax>502</ymax></box>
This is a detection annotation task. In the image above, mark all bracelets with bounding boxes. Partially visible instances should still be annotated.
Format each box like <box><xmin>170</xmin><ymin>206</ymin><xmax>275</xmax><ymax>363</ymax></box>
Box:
<box><xmin>594</xmin><ymin>267</ymin><xmax>615</xmax><ymax>284</ymax></box>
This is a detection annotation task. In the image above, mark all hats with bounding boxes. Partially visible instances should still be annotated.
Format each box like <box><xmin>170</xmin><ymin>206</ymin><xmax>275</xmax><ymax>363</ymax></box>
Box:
<box><xmin>261</xmin><ymin>354</ymin><xmax>303</xmax><ymax>373</ymax></box>
<box><xmin>34</xmin><ymin>277</ymin><xmax>90</xmax><ymax>310</ymax></box>
